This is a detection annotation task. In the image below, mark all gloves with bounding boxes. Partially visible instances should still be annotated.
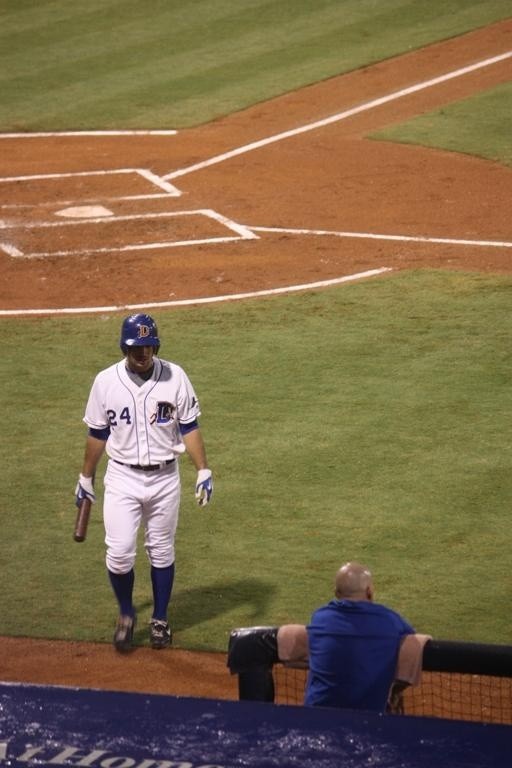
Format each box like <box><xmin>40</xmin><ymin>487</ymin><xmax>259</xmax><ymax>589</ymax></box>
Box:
<box><xmin>72</xmin><ymin>472</ymin><xmax>96</xmax><ymax>507</ymax></box>
<box><xmin>194</xmin><ymin>468</ymin><xmax>213</xmax><ymax>507</ymax></box>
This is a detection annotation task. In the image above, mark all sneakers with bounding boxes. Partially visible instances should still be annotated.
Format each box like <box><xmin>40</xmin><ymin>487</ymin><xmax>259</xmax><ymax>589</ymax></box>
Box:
<box><xmin>112</xmin><ymin>614</ymin><xmax>137</xmax><ymax>652</ymax></box>
<box><xmin>149</xmin><ymin>617</ymin><xmax>172</xmax><ymax>650</ymax></box>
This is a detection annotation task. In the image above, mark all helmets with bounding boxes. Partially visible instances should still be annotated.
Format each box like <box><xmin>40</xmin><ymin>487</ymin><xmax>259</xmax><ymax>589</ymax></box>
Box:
<box><xmin>120</xmin><ymin>312</ymin><xmax>161</xmax><ymax>357</ymax></box>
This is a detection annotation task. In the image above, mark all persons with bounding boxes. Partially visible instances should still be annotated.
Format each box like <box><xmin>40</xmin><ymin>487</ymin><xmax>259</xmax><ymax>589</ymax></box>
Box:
<box><xmin>73</xmin><ymin>312</ymin><xmax>214</xmax><ymax>651</ymax></box>
<box><xmin>302</xmin><ymin>562</ymin><xmax>418</xmax><ymax>715</ymax></box>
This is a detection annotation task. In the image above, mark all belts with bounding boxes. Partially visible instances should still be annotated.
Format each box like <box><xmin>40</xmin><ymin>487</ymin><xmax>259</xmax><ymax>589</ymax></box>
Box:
<box><xmin>113</xmin><ymin>459</ymin><xmax>176</xmax><ymax>471</ymax></box>
